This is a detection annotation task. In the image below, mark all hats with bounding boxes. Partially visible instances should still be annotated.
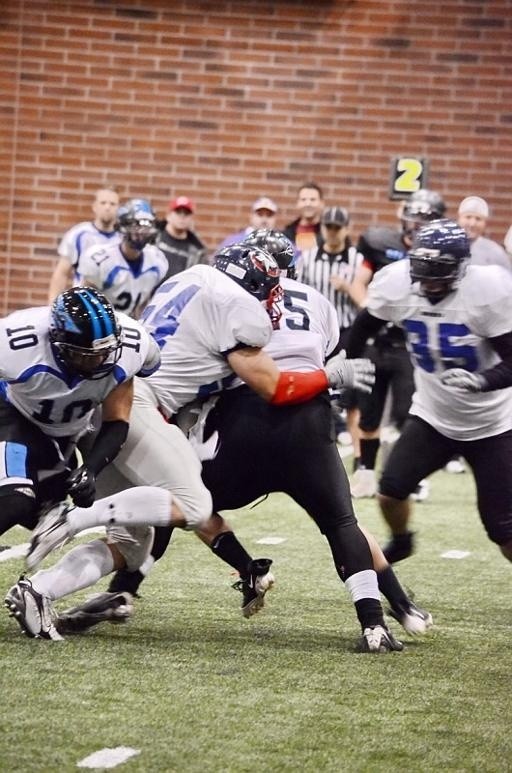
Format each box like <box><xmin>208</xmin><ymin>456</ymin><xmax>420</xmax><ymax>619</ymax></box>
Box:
<box><xmin>459</xmin><ymin>195</ymin><xmax>488</xmax><ymax>217</ymax></box>
<box><xmin>252</xmin><ymin>196</ymin><xmax>277</xmax><ymax>213</ymax></box>
<box><xmin>321</xmin><ymin>206</ymin><xmax>349</xmax><ymax>226</ymax></box>
<box><xmin>169</xmin><ymin>196</ymin><xmax>194</xmax><ymax>213</ymax></box>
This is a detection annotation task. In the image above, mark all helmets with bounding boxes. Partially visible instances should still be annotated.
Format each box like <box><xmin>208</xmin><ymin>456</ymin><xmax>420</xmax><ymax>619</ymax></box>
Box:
<box><xmin>397</xmin><ymin>188</ymin><xmax>471</xmax><ymax>297</ymax></box>
<box><xmin>212</xmin><ymin>227</ymin><xmax>298</xmax><ymax>322</ymax></box>
<box><xmin>113</xmin><ymin>199</ymin><xmax>158</xmax><ymax>250</ymax></box>
<box><xmin>48</xmin><ymin>285</ymin><xmax>125</xmax><ymax>380</ymax></box>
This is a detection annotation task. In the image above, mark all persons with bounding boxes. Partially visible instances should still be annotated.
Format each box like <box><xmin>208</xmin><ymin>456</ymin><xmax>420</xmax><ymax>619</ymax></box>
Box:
<box><xmin>335</xmin><ymin>217</ymin><xmax>510</xmax><ymax>565</ymax></box>
<box><xmin>1</xmin><ymin>227</ymin><xmax>434</xmax><ymax>653</ymax></box>
<box><xmin>50</xmin><ymin>184</ymin><xmax>511</xmax><ymax>500</ymax></box>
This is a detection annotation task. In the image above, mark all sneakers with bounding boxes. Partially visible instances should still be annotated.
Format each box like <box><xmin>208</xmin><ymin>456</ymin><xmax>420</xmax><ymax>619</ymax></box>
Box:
<box><xmin>356</xmin><ymin>623</ymin><xmax>403</xmax><ymax>653</ymax></box>
<box><xmin>58</xmin><ymin>591</ymin><xmax>134</xmax><ymax>632</ymax></box>
<box><xmin>337</xmin><ymin>422</ymin><xmax>466</xmax><ymax>502</ymax></box>
<box><xmin>25</xmin><ymin>499</ymin><xmax>74</xmax><ymax>572</ymax></box>
<box><xmin>5</xmin><ymin>575</ymin><xmax>65</xmax><ymax>642</ymax></box>
<box><xmin>386</xmin><ymin>584</ymin><xmax>433</xmax><ymax>635</ymax></box>
<box><xmin>231</xmin><ymin>559</ymin><xmax>274</xmax><ymax>619</ymax></box>
<box><xmin>382</xmin><ymin>531</ymin><xmax>416</xmax><ymax>563</ymax></box>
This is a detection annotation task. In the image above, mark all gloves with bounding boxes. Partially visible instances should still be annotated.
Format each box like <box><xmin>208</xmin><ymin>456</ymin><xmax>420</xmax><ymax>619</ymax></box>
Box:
<box><xmin>63</xmin><ymin>467</ymin><xmax>96</xmax><ymax>507</ymax></box>
<box><xmin>321</xmin><ymin>349</ymin><xmax>376</xmax><ymax>394</ymax></box>
<box><xmin>438</xmin><ymin>367</ymin><xmax>482</xmax><ymax>392</ymax></box>
